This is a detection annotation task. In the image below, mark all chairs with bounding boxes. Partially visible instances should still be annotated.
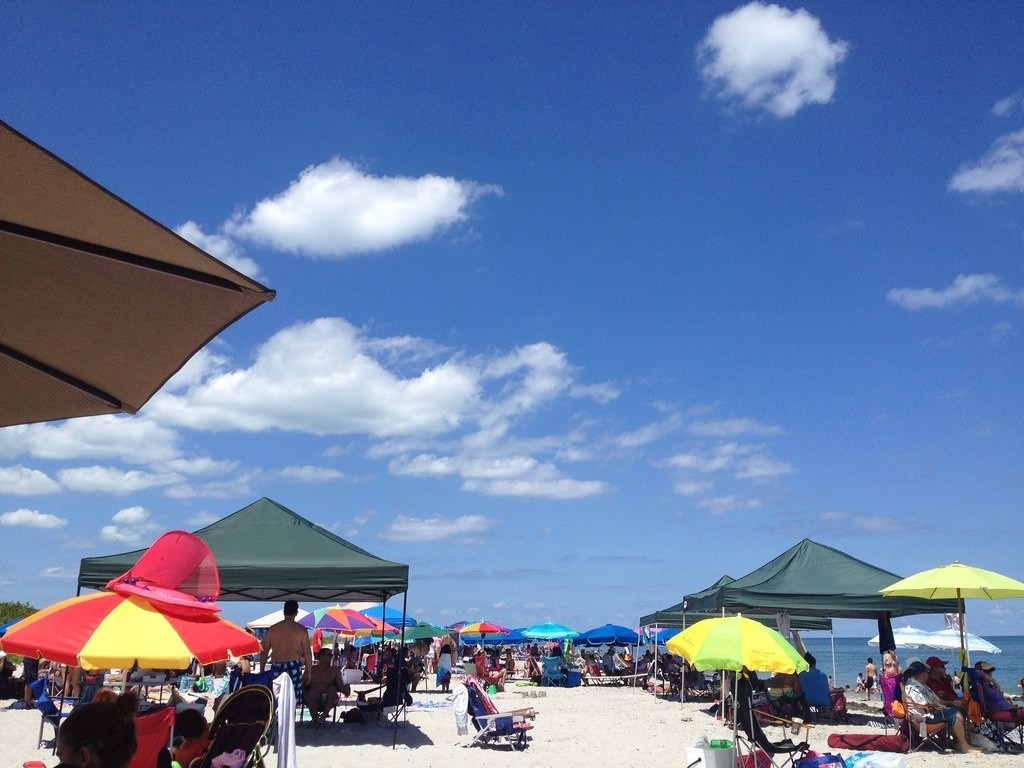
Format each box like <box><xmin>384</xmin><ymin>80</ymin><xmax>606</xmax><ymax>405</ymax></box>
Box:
<box><xmin>0</xmin><ymin>645</ymin><xmax>1024</xmax><ymax>768</ymax></box>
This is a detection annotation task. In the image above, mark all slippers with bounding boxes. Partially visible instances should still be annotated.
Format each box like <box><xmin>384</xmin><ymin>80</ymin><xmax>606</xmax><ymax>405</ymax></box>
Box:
<box><xmin>993</xmin><ymin>743</ymin><xmax>1018</xmax><ymax>754</ymax></box>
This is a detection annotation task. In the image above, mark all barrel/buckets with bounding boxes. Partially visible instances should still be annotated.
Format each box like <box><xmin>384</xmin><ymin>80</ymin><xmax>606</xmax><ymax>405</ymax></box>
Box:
<box><xmin>487</xmin><ymin>684</ymin><xmax>497</xmax><ymax>694</ymax></box>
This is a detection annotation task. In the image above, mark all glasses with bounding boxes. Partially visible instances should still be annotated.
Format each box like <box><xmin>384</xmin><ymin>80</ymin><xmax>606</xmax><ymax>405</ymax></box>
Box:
<box><xmin>323</xmin><ymin>653</ymin><xmax>333</xmax><ymax>658</ymax></box>
<box><xmin>937</xmin><ymin>665</ymin><xmax>944</xmax><ymax>668</ymax></box>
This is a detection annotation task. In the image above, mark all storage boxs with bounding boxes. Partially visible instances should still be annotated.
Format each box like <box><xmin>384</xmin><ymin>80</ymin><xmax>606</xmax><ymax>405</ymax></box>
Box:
<box><xmin>143</xmin><ymin>672</ymin><xmax>164</xmax><ymax>683</ymax></box>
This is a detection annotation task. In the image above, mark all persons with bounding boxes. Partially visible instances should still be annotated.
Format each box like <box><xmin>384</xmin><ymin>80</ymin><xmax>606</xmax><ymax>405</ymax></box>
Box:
<box><xmin>0</xmin><ymin>601</ymin><xmax>1024</xmax><ymax>753</ymax></box>
<box><xmin>53</xmin><ymin>692</ymin><xmax>141</xmax><ymax>768</ymax></box>
<box><xmin>157</xmin><ymin>709</ymin><xmax>209</xmax><ymax>768</ymax></box>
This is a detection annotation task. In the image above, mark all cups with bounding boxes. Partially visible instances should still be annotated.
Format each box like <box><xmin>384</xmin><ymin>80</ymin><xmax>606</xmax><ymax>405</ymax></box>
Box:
<box><xmin>794</xmin><ymin>718</ymin><xmax>803</xmax><ymax>724</ymax></box>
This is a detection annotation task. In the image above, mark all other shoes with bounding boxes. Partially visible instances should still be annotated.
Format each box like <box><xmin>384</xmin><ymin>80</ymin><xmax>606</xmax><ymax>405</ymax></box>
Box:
<box><xmin>582</xmin><ymin>682</ymin><xmax>586</xmax><ymax>685</ymax></box>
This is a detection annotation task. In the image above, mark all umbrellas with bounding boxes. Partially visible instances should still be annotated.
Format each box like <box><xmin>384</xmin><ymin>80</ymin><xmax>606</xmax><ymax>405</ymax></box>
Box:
<box><xmin>0</xmin><ymin>590</ymin><xmax>265</xmax><ymax>762</ymax></box>
<box><xmin>0</xmin><ymin>120</ymin><xmax>278</xmax><ymax>432</ymax></box>
<box><xmin>298</xmin><ymin>604</ymin><xmax>683</xmax><ymax>660</ymax></box>
<box><xmin>665</xmin><ymin>612</ymin><xmax>810</xmax><ymax>768</ymax></box>
<box><xmin>867</xmin><ymin>561</ymin><xmax>1024</xmax><ymax>671</ymax></box>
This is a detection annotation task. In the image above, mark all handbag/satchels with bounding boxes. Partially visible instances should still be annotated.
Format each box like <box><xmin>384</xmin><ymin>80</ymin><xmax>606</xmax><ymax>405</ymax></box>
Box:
<box><xmin>792</xmin><ymin>752</ymin><xmax>848</xmax><ymax>768</ymax></box>
<box><xmin>382</xmin><ymin>667</ymin><xmax>413</xmax><ymax>707</ymax></box>
<box><xmin>340</xmin><ymin>708</ymin><xmax>364</xmax><ymax>726</ymax></box>
<box><xmin>193</xmin><ymin>678</ymin><xmax>209</xmax><ymax>692</ymax></box>
<box><xmin>739</xmin><ymin>750</ymin><xmax>774</xmax><ymax>768</ymax></box>
<box><xmin>831</xmin><ymin>689</ymin><xmax>846</xmax><ymax>713</ymax></box>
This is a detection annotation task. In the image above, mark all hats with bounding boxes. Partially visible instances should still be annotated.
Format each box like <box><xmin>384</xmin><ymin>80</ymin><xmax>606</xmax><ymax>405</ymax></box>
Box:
<box><xmin>583</xmin><ymin>651</ymin><xmax>592</xmax><ymax>655</ymax></box>
<box><xmin>610</xmin><ymin>647</ymin><xmax>618</xmax><ymax>653</ymax></box>
<box><xmin>975</xmin><ymin>662</ymin><xmax>995</xmax><ymax>671</ymax></box>
<box><xmin>926</xmin><ymin>657</ymin><xmax>948</xmax><ymax>667</ymax></box>
<box><xmin>345</xmin><ymin>640</ymin><xmax>349</xmax><ymax>644</ymax></box>
<box><xmin>909</xmin><ymin>661</ymin><xmax>932</xmax><ymax>672</ymax></box>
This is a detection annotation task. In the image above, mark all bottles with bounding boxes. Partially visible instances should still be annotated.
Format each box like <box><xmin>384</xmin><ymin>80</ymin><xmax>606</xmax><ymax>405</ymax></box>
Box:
<box><xmin>711</xmin><ymin>740</ymin><xmax>733</xmax><ymax>748</ymax></box>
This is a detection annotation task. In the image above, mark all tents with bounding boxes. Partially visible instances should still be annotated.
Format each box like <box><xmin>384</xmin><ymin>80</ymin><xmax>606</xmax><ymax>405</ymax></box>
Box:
<box><xmin>76</xmin><ymin>497</ymin><xmax>409</xmax><ymax>751</ymax></box>
<box><xmin>245</xmin><ymin>606</ymin><xmax>311</xmax><ymax>629</ymax></box>
<box><xmin>633</xmin><ymin>538</ymin><xmax>971</xmax><ymax>735</ymax></box>
